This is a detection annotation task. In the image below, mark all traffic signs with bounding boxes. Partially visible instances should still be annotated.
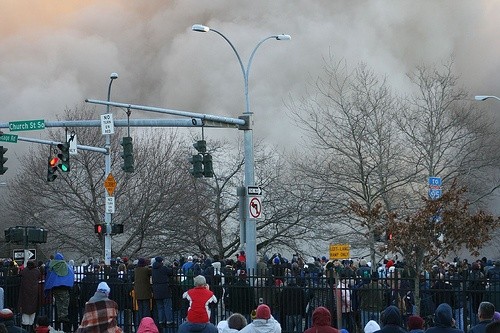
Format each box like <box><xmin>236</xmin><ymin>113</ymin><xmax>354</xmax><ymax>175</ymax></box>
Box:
<box><xmin>13</xmin><ymin>249</ymin><xmax>37</xmax><ymax>261</ymax></box>
<box><xmin>248</xmin><ymin>186</ymin><xmax>262</xmax><ymax>196</ymax></box>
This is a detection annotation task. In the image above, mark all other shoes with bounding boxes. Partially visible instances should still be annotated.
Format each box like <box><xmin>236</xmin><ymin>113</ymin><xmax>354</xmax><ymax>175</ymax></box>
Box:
<box><xmin>166</xmin><ymin>321</ymin><xmax>177</xmax><ymax>328</ymax></box>
<box><xmin>158</xmin><ymin>323</ymin><xmax>165</xmax><ymax>328</ymax></box>
<box><xmin>57</xmin><ymin>317</ymin><xmax>71</xmax><ymax>322</ymax></box>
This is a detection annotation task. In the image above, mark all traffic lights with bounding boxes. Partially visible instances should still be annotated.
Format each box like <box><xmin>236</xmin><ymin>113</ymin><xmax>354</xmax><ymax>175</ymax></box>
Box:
<box><xmin>94</xmin><ymin>223</ymin><xmax>106</xmax><ymax>234</ymax></box>
<box><xmin>57</xmin><ymin>143</ymin><xmax>70</xmax><ymax>173</ymax></box>
<box><xmin>189</xmin><ymin>140</ymin><xmax>213</xmax><ymax>178</ymax></box>
<box><xmin>120</xmin><ymin>135</ymin><xmax>134</xmax><ymax>173</ymax></box>
<box><xmin>113</xmin><ymin>223</ymin><xmax>124</xmax><ymax>233</ymax></box>
<box><xmin>47</xmin><ymin>156</ymin><xmax>57</xmax><ymax>182</ymax></box>
<box><xmin>0</xmin><ymin>145</ymin><xmax>9</xmax><ymax>175</ymax></box>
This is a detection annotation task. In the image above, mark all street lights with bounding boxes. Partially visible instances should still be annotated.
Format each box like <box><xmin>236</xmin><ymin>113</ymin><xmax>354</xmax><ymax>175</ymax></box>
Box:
<box><xmin>192</xmin><ymin>23</ymin><xmax>291</xmax><ymax>282</ymax></box>
<box><xmin>105</xmin><ymin>73</ymin><xmax>119</xmax><ymax>265</ymax></box>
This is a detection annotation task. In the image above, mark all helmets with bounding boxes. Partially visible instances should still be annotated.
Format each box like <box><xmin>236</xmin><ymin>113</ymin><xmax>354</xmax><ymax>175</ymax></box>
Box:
<box><xmin>0</xmin><ymin>308</ymin><xmax>13</xmax><ymax>321</ymax></box>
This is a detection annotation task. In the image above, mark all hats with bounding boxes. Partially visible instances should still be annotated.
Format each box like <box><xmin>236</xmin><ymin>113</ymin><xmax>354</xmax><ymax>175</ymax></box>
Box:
<box><xmin>360</xmin><ymin>259</ymin><xmax>367</xmax><ymax>266</ymax></box>
<box><xmin>122</xmin><ymin>257</ymin><xmax>128</xmax><ymax>261</ymax></box>
<box><xmin>35</xmin><ymin>316</ymin><xmax>50</xmax><ymax>326</ymax></box>
<box><xmin>256</xmin><ymin>305</ymin><xmax>270</xmax><ymax>319</ymax></box>
<box><xmin>138</xmin><ymin>258</ymin><xmax>145</xmax><ymax>263</ymax></box>
<box><xmin>155</xmin><ymin>257</ymin><xmax>162</xmax><ymax>262</ymax></box>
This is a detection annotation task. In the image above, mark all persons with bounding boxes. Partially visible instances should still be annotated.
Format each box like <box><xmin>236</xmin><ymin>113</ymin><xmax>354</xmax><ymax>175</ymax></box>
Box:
<box><xmin>182</xmin><ymin>275</ymin><xmax>217</xmax><ymax>323</ymax></box>
<box><xmin>44</xmin><ymin>253</ymin><xmax>74</xmax><ymax>322</ymax></box>
<box><xmin>151</xmin><ymin>256</ymin><xmax>178</xmax><ymax>327</ymax></box>
<box><xmin>0</xmin><ymin>251</ymin><xmax>500</xmax><ymax>333</ymax></box>
